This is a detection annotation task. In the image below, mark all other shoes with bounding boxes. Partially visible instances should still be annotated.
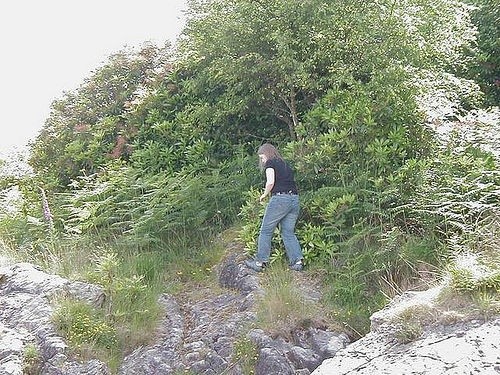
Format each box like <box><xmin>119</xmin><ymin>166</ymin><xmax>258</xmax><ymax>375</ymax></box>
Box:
<box><xmin>245</xmin><ymin>260</ymin><xmax>262</xmax><ymax>272</ymax></box>
<box><xmin>288</xmin><ymin>264</ymin><xmax>303</xmax><ymax>271</ymax></box>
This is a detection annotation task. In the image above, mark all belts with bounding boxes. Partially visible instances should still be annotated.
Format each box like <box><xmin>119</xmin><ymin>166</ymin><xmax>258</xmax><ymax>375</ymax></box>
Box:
<box><xmin>273</xmin><ymin>192</ymin><xmax>298</xmax><ymax>195</ymax></box>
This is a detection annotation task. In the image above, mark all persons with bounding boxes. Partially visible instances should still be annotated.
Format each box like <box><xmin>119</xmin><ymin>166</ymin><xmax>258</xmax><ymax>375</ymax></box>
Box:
<box><xmin>244</xmin><ymin>143</ymin><xmax>304</xmax><ymax>272</ymax></box>
